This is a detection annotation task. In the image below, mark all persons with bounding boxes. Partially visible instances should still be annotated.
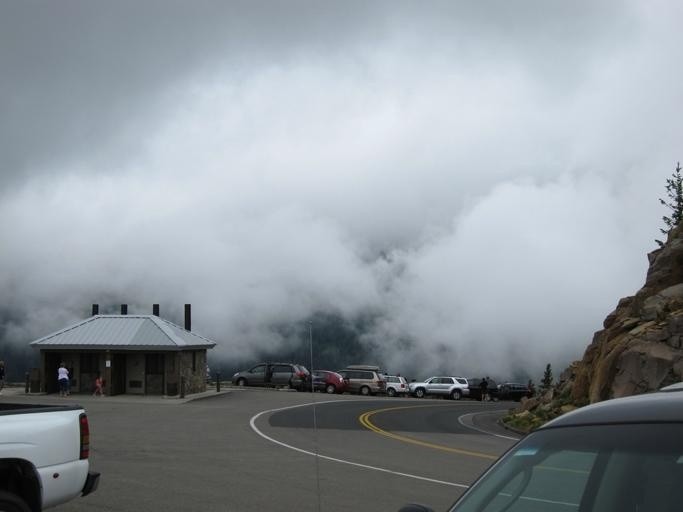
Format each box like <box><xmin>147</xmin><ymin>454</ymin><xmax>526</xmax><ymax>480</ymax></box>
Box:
<box><xmin>527</xmin><ymin>379</ymin><xmax>535</xmax><ymax>399</ymax></box>
<box><xmin>479</xmin><ymin>377</ymin><xmax>487</xmax><ymax>401</ymax></box>
<box><xmin>57</xmin><ymin>362</ymin><xmax>69</xmax><ymax>398</ymax></box>
<box><xmin>0</xmin><ymin>360</ymin><xmax>4</xmax><ymax>393</ymax></box>
<box><xmin>92</xmin><ymin>371</ymin><xmax>104</xmax><ymax>398</ymax></box>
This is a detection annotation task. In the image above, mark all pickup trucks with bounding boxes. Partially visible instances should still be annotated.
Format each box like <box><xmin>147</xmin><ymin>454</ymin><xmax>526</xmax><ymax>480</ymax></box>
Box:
<box><xmin>0</xmin><ymin>400</ymin><xmax>100</xmax><ymax>512</ymax></box>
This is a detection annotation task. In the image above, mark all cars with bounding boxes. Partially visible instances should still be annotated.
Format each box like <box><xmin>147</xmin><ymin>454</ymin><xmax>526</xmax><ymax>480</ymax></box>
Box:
<box><xmin>310</xmin><ymin>370</ymin><xmax>346</xmax><ymax>394</ymax></box>
<box><xmin>231</xmin><ymin>362</ymin><xmax>312</xmax><ymax>391</ymax></box>
<box><xmin>471</xmin><ymin>378</ymin><xmax>498</xmax><ymax>401</ymax></box>
<box><xmin>385</xmin><ymin>375</ymin><xmax>409</xmax><ymax>396</ymax></box>
<box><xmin>493</xmin><ymin>383</ymin><xmax>531</xmax><ymax>401</ymax></box>
<box><xmin>399</xmin><ymin>381</ymin><xmax>682</xmax><ymax>512</ymax></box>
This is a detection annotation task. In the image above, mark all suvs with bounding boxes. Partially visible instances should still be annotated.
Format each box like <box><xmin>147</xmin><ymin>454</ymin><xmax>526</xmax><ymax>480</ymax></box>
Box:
<box><xmin>337</xmin><ymin>370</ymin><xmax>383</xmax><ymax>395</ymax></box>
<box><xmin>408</xmin><ymin>376</ymin><xmax>470</xmax><ymax>400</ymax></box>
